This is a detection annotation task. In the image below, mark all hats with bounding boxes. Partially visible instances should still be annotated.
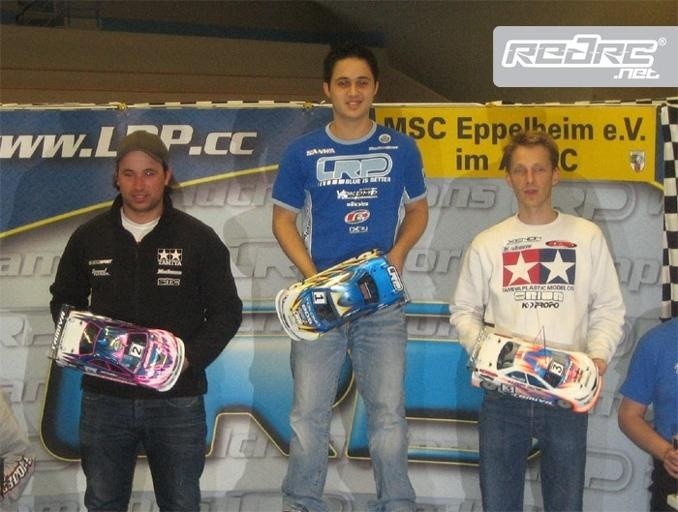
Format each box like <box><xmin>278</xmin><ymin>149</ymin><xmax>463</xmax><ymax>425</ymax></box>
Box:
<box><xmin>114</xmin><ymin>129</ymin><xmax>171</xmax><ymax>166</ymax></box>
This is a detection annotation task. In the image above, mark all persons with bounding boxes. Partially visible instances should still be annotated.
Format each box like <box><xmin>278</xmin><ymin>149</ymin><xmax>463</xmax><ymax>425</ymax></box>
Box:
<box><xmin>48</xmin><ymin>131</ymin><xmax>241</xmax><ymax>511</ymax></box>
<box><xmin>450</xmin><ymin>128</ymin><xmax>627</xmax><ymax>512</ymax></box>
<box><xmin>268</xmin><ymin>39</ymin><xmax>431</xmax><ymax>511</ymax></box>
<box><xmin>617</xmin><ymin>311</ymin><xmax>678</xmax><ymax>512</ymax></box>
